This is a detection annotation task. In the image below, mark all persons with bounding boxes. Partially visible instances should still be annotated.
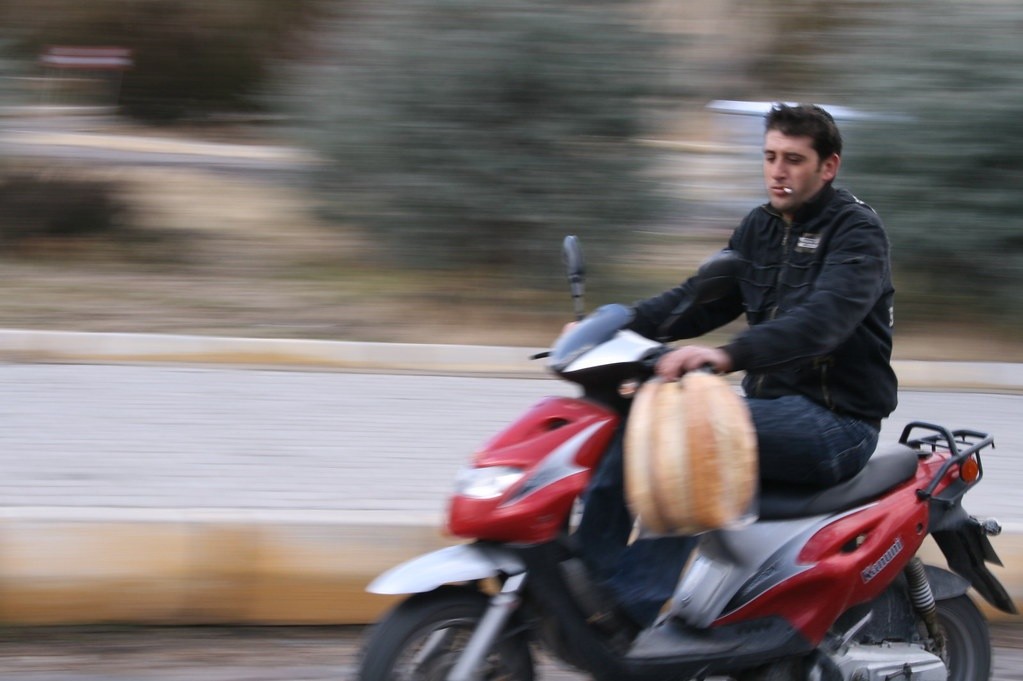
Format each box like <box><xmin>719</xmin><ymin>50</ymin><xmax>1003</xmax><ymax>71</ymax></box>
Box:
<box><xmin>560</xmin><ymin>103</ymin><xmax>898</xmax><ymax>655</ymax></box>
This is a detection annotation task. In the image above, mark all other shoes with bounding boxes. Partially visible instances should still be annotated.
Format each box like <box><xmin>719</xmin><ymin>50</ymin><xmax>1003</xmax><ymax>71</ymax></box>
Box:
<box><xmin>559</xmin><ymin>561</ymin><xmax>634</xmax><ymax>656</ymax></box>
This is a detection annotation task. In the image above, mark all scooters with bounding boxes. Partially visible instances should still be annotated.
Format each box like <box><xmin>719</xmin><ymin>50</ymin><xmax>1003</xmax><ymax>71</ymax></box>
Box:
<box><xmin>353</xmin><ymin>233</ymin><xmax>1021</xmax><ymax>681</ymax></box>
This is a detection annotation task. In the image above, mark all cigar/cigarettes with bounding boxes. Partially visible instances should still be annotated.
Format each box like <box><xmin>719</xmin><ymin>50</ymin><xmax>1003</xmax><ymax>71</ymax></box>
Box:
<box><xmin>782</xmin><ymin>188</ymin><xmax>792</xmax><ymax>194</ymax></box>
